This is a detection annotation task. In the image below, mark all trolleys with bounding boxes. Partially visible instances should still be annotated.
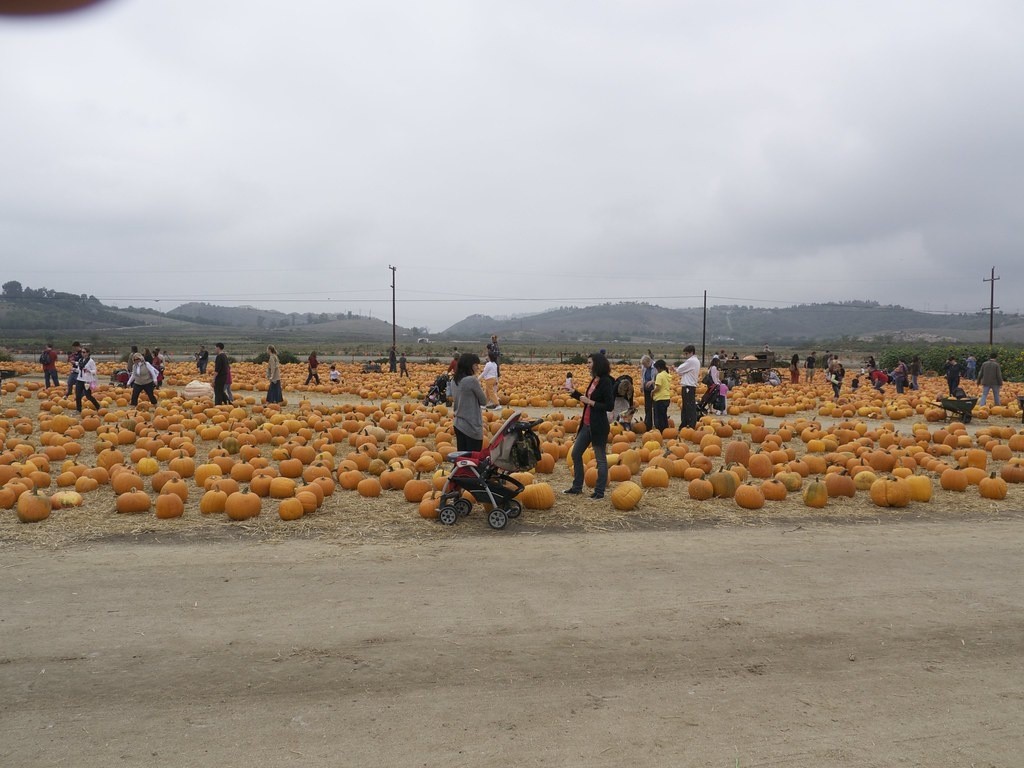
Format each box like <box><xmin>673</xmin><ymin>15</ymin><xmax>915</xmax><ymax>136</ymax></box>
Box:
<box><xmin>925</xmin><ymin>395</ymin><xmax>979</xmax><ymax>423</ymax></box>
<box><xmin>1014</xmin><ymin>396</ymin><xmax>1024</xmax><ymax>424</ymax></box>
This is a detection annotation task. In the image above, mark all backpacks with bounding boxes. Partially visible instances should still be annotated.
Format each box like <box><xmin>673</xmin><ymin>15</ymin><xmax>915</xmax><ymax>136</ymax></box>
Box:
<box><xmin>39</xmin><ymin>350</ymin><xmax>54</xmax><ymax>364</ymax></box>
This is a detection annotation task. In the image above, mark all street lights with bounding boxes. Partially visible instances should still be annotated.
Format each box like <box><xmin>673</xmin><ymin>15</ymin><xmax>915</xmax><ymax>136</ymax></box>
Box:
<box><xmin>388</xmin><ymin>265</ymin><xmax>396</xmax><ymax>350</ymax></box>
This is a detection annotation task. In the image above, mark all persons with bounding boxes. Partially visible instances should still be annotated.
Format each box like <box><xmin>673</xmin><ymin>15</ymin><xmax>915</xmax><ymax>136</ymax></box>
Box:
<box><xmin>672</xmin><ymin>344</ymin><xmax>701</xmax><ymax>429</ymax></box>
<box><xmin>708</xmin><ymin>349</ymin><xmax>781</xmax><ymax>415</ymax></box>
<box><xmin>851</xmin><ymin>374</ymin><xmax>860</xmax><ymax>393</ymax></box>
<box><xmin>883</xmin><ymin>357</ymin><xmax>908</xmax><ymax>394</ymax></box>
<box><xmin>867</xmin><ymin>363</ymin><xmax>888</xmax><ymax>394</ymax></box>
<box><xmin>266</xmin><ymin>344</ymin><xmax>285</xmax><ymax>402</ymax></box>
<box><xmin>565</xmin><ymin>353</ymin><xmax>616</xmax><ymax>499</ymax></box>
<box><xmin>447</xmin><ymin>333</ymin><xmax>501</xmax><ymax>454</ymax></box>
<box><xmin>827</xmin><ymin>353</ymin><xmax>841</xmax><ymax>376</ymax></box>
<box><xmin>43</xmin><ymin>341</ymin><xmax>235</xmax><ymax>412</ymax></box>
<box><xmin>911</xmin><ymin>355</ymin><xmax>919</xmax><ymax>390</ymax></box>
<box><xmin>390</xmin><ymin>346</ymin><xmax>410</xmax><ymax>377</ymax></box>
<box><xmin>867</xmin><ymin>356</ymin><xmax>875</xmax><ymax>380</ymax></box>
<box><xmin>831</xmin><ymin>363</ymin><xmax>845</xmax><ymax>399</ymax></box>
<box><xmin>805</xmin><ymin>351</ymin><xmax>816</xmax><ymax>383</ymax></box>
<box><xmin>304</xmin><ymin>351</ymin><xmax>341</xmax><ymax>384</ymax></box>
<box><xmin>943</xmin><ymin>356</ymin><xmax>967</xmax><ymax>397</ymax></box>
<box><xmin>963</xmin><ymin>353</ymin><xmax>976</xmax><ymax>379</ymax></box>
<box><xmin>975</xmin><ymin>352</ymin><xmax>1004</xmax><ymax>408</ymax></box>
<box><xmin>789</xmin><ymin>354</ymin><xmax>800</xmax><ymax>384</ymax></box>
<box><xmin>822</xmin><ymin>350</ymin><xmax>829</xmax><ymax>382</ymax></box>
<box><xmin>860</xmin><ymin>366</ymin><xmax>865</xmax><ymax>375</ymax></box>
<box><xmin>610</xmin><ymin>355</ymin><xmax>674</xmax><ymax>431</ymax></box>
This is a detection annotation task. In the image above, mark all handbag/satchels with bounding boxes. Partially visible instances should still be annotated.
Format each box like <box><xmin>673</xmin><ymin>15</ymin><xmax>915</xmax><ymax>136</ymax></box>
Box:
<box><xmin>511</xmin><ymin>422</ymin><xmax>541</xmax><ymax>472</ymax></box>
<box><xmin>145</xmin><ymin>362</ymin><xmax>160</xmax><ymax>379</ymax></box>
<box><xmin>702</xmin><ymin>374</ymin><xmax>714</xmax><ymax>385</ymax></box>
<box><xmin>311</xmin><ymin>368</ymin><xmax>317</xmax><ymax>375</ymax></box>
<box><xmin>446</xmin><ymin>381</ymin><xmax>452</xmax><ymax>397</ymax></box>
<box><xmin>713</xmin><ymin>389</ymin><xmax>725</xmax><ymax>411</ymax></box>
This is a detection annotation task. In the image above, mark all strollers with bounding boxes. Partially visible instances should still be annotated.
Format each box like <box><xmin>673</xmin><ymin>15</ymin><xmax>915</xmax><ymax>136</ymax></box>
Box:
<box><xmin>695</xmin><ymin>383</ymin><xmax>721</xmax><ymax>423</ymax></box>
<box><xmin>435</xmin><ymin>412</ymin><xmax>544</xmax><ymax>530</ymax></box>
<box><xmin>422</xmin><ymin>372</ymin><xmax>456</xmax><ymax>406</ymax></box>
<box><xmin>606</xmin><ymin>374</ymin><xmax>638</xmax><ymax>430</ymax></box>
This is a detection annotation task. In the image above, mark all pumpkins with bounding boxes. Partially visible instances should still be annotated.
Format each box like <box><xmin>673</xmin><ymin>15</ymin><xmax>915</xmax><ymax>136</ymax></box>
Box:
<box><xmin>0</xmin><ymin>362</ymin><xmax>1024</xmax><ymax>522</ymax></box>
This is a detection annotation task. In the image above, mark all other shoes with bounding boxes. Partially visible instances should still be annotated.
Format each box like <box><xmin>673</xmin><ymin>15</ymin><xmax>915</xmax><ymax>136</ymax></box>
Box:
<box><xmin>481</xmin><ymin>405</ymin><xmax>487</xmax><ymax>409</ymax></box>
<box><xmin>493</xmin><ymin>405</ymin><xmax>502</xmax><ymax>410</ymax></box>
<box><xmin>587</xmin><ymin>493</ymin><xmax>604</xmax><ymax>500</ymax></box>
<box><xmin>563</xmin><ymin>488</ymin><xmax>582</xmax><ymax>495</ymax></box>
